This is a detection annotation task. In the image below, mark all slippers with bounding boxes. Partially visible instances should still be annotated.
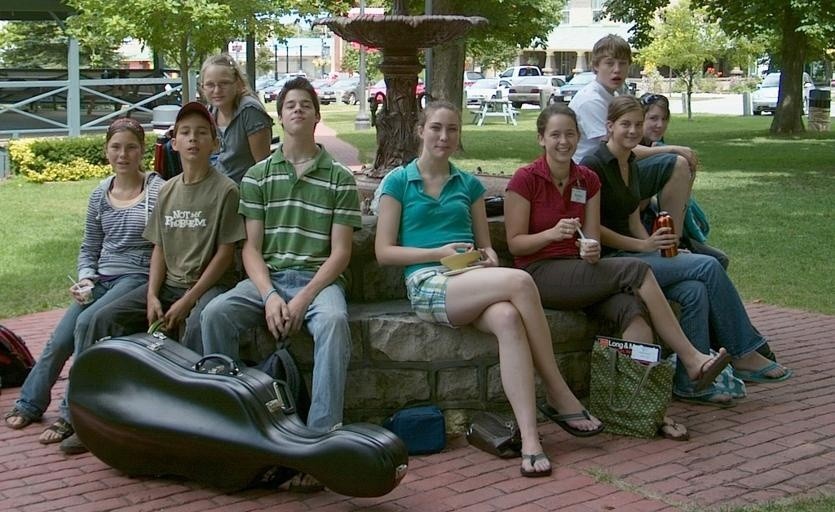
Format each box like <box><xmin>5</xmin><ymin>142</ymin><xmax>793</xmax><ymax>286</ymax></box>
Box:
<box><xmin>659</xmin><ymin>419</ymin><xmax>689</xmax><ymax>441</ymax></box>
<box><xmin>537</xmin><ymin>396</ymin><xmax>604</xmax><ymax>437</ymax></box>
<box><xmin>289</xmin><ymin>472</ymin><xmax>324</xmax><ymax>493</ymax></box>
<box><xmin>734</xmin><ymin>360</ymin><xmax>793</xmax><ymax>382</ymax></box>
<box><xmin>672</xmin><ymin>389</ymin><xmax>737</xmax><ymax>408</ymax></box>
<box><xmin>692</xmin><ymin>354</ymin><xmax>733</xmax><ymax>393</ymax></box>
<box><xmin>520</xmin><ymin>452</ymin><xmax>551</xmax><ymax>477</ymax></box>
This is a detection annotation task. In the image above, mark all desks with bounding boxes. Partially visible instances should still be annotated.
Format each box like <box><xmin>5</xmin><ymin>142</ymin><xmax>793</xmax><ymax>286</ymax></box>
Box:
<box><xmin>473</xmin><ymin>99</ymin><xmax>517</xmax><ymax>128</ymax></box>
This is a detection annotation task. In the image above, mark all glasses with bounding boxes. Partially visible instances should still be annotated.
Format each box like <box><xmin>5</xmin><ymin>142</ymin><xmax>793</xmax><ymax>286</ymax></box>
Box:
<box><xmin>203</xmin><ymin>77</ymin><xmax>238</xmax><ymax>90</ymax></box>
<box><xmin>639</xmin><ymin>96</ymin><xmax>668</xmax><ymax>105</ymax></box>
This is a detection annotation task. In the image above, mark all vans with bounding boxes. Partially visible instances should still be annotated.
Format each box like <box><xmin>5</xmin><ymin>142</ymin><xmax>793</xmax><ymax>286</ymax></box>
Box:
<box><xmin>279</xmin><ymin>73</ymin><xmax>307</xmax><ymax>80</ymax></box>
<box><xmin>464</xmin><ymin>71</ymin><xmax>485</xmax><ymax>93</ymax></box>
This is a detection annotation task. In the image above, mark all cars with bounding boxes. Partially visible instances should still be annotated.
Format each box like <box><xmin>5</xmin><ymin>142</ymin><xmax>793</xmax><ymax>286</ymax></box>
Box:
<box><xmin>508</xmin><ymin>76</ymin><xmax>566</xmax><ymax>109</ymax></box>
<box><xmin>553</xmin><ymin>72</ymin><xmax>630</xmax><ymax>107</ymax></box>
<box><xmin>369</xmin><ymin>79</ymin><xmax>425</xmax><ymax>103</ymax></box>
<box><xmin>464</xmin><ymin>79</ymin><xmax>513</xmax><ymax>109</ymax></box>
<box><xmin>751</xmin><ymin>72</ymin><xmax>815</xmax><ymax>116</ymax></box>
<box><xmin>263</xmin><ymin>80</ymin><xmax>290</xmax><ymax>102</ymax></box>
<box><xmin>318</xmin><ymin>80</ymin><xmax>361</xmax><ymax>105</ymax></box>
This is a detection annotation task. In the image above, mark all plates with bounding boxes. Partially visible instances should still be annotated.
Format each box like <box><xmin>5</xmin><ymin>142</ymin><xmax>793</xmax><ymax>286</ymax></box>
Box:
<box><xmin>443</xmin><ymin>265</ymin><xmax>485</xmax><ymax>276</ymax></box>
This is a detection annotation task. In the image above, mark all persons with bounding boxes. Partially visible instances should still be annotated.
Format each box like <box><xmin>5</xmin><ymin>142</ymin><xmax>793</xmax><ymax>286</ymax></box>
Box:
<box><xmin>200</xmin><ymin>78</ymin><xmax>363</xmax><ymax>491</ymax></box>
<box><xmin>580</xmin><ymin>95</ymin><xmax>792</xmax><ymax>409</ymax></box>
<box><xmin>567</xmin><ymin>34</ymin><xmax>697</xmax><ymax>252</ymax></box>
<box><xmin>504</xmin><ymin>101</ymin><xmax>731</xmax><ymax>442</ymax></box>
<box><xmin>60</xmin><ymin>101</ymin><xmax>248</xmax><ymax>455</ymax></box>
<box><xmin>3</xmin><ymin>119</ymin><xmax>168</xmax><ymax>444</ymax></box>
<box><xmin>166</xmin><ymin>54</ymin><xmax>272</xmax><ymax>183</ymax></box>
<box><xmin>638</xmin><ymin>92</ymin><xmax>729</xmax><ymax>269</ymax></box>
<box><xmin>374</xmin><ymin>100</ymin><xmax>606</xmax><ymax>478</ymax></box>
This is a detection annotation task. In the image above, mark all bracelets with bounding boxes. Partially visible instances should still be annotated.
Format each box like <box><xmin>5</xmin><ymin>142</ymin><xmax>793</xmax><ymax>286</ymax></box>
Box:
<box><xmin>262</xmin><ymin>289</ymin><xmax>275</xmax><ymax>307</ymax></box>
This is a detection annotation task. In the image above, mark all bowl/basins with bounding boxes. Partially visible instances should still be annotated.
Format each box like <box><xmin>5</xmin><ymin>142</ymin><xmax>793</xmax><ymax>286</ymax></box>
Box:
<box><xmin>440</xmin><ymin>249</ymin><xmax>482</xmax><ymax>269</ymax></box>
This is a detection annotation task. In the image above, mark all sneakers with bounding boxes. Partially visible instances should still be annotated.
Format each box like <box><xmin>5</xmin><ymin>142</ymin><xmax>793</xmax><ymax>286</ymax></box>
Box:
<box><xmin>59</xmin><ymin>432</ymin><xmax>88</xmax><ymax>453</ymax></box>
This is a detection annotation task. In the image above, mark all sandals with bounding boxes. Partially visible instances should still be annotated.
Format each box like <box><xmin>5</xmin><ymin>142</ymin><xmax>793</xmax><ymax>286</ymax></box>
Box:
<box><xmin>38</xmin><ymin>417</ymin><xmax>74</xmax><ymax>443</ymax></box>
<box><xmin>6</xmin><ymin>408</ymin><xmax>32</xmax><ymax>428</ymax></box>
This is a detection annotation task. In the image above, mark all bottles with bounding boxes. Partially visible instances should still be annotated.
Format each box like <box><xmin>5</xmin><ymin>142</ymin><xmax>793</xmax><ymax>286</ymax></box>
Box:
<box><xmin>657</xmin><ymin>212</ymin><xmax>677</xmax><ymax>257</ymax></box>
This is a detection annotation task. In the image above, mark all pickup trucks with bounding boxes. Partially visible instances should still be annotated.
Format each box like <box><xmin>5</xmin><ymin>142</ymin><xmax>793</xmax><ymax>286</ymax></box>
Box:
<box><xmin>495</xmin><ymin>66</ymin><xmax>566</xmax><ymax>90</ymax></box>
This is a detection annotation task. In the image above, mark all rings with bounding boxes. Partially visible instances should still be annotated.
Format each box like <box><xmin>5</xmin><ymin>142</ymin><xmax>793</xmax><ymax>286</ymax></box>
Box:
<box><xmin>566</xmin><ymin>228</ymin><xmax>571</xmax><ymax>234</ymax></box>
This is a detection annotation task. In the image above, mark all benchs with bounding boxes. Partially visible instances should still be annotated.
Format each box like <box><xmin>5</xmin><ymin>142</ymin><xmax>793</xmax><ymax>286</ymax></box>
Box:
<box><xmin>471</xmin><ymin>111</ymin><xmax>520</xmax><ymax>121</ymax></box>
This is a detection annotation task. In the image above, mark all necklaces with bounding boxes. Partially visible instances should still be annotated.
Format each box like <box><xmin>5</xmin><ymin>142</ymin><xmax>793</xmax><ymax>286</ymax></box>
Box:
<box><xmin>619</xmin><ymin>157</ymin><xmax>629</xmax><ymax>171</ymax></box>
<box><xmin>291</xmin><ymin>144</ymin><xmax>322</xmax><ymax>169</ymax></box>
<box><xmin>551</xmin><ymin>170</ymin><xmax>570</xmax><ymax>187</ymax></box>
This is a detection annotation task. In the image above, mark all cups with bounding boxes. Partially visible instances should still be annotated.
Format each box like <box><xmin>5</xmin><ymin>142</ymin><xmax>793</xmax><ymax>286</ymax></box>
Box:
<box><xmin>71</xmin><ymin>284</ymin><xmax>95</xmax><ymax>305</ymax></box>
<box><xmin>577</xmin><ymin>239</ymin><xmax>598</xmax><ymax>261</ymax></box>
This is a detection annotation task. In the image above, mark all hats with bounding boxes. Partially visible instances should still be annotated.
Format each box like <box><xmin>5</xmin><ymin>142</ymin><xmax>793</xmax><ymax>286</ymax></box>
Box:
<box><xmin>176</xmin><ymin>102</ymin><xmax>216</xmax><ymax>138</ymax></box>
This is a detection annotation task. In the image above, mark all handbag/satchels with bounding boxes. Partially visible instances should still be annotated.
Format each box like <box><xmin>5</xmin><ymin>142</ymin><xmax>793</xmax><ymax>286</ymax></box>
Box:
<box><xmin>588</xmin><ymin>341</ymin><xmax>677</xmax><ymax>441</ymax></box>
<box><xmin>466</xmin><ymin>412</ymin><xmax>544</xmax><ymax>461</ymax></box>
<box><xmin>381</xmin><ymin>402</ymin><xmax>446</xmax><ymax>455</ymax></box>
<box><xmin>666</xmin><ymin>348</ymin><xmax>746</xmax><ymax>398</ymax></box>
